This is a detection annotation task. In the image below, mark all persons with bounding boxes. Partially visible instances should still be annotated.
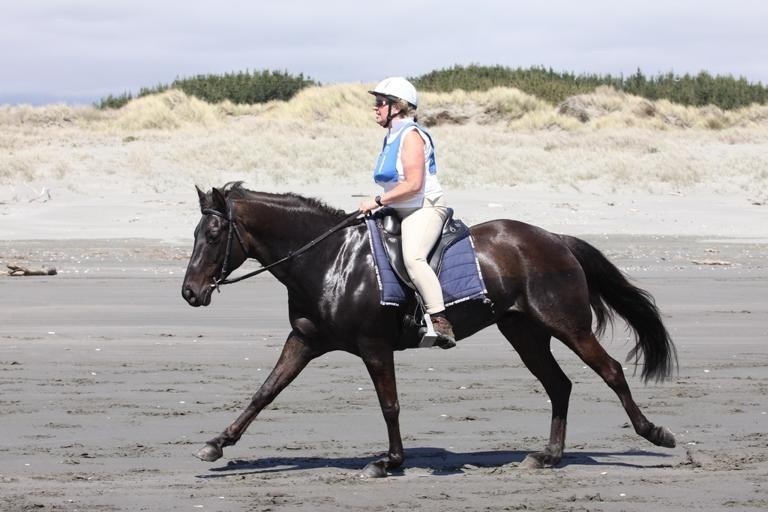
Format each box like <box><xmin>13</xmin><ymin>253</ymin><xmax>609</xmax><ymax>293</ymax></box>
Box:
<box><xmin>359</xmin><ymin>77</ymin><xmax>456</xmax><ymax>350</ymax></box>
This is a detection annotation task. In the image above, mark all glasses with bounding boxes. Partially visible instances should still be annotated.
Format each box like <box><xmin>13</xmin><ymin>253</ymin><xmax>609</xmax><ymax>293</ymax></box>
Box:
<box><xmin>375</xmin><ymin>99</ymin><xmax>392</xmax><ymax>107</ymax></box>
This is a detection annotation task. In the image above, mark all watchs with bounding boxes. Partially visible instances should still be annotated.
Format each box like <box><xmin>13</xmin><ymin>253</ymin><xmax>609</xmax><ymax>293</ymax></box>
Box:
<box><xmin>375</xmin><ymin>195</ymin><xmax>383</xmax><ymax>206</ymax></box>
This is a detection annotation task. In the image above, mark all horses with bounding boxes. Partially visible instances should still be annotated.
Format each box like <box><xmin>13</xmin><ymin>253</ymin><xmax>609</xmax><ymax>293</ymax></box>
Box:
<box><xmin>181</xmin><ymin>181</ymin><xmax>680</xmax><ymax>478</ymax></box>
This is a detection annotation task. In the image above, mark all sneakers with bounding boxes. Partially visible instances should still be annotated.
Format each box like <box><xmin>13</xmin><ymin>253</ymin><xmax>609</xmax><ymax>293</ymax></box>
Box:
<box><xmin>417</xmin><ymin>317</ymin><xmax>456</xmax><ymax>349</ymax></box>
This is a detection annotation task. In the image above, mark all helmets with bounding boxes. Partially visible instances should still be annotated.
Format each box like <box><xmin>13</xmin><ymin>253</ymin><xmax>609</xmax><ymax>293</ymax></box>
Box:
<box><xmin>368</xmin><ymin>76</ymin><xmax>418</xmax><ymax>108</ymax></box>
